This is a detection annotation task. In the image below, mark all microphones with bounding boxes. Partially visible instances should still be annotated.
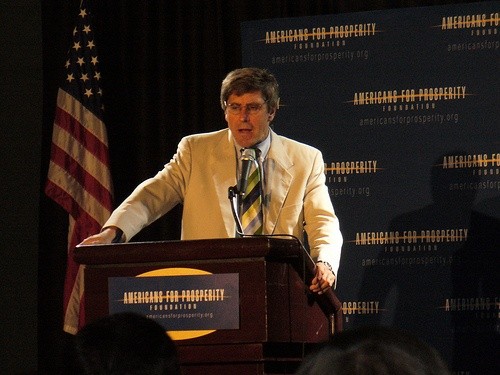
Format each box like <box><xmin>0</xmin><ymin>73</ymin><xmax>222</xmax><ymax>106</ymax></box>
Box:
<box><xmin>240</xmin><ymin>149</ymin><xmax>255</xmax><ymax>197</ymax></box>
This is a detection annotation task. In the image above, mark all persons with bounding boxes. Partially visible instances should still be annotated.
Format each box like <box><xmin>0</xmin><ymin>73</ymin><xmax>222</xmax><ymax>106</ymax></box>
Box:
<box><xmin>58</xmin><ymin>311</ymin><xmax>183</xmax><ymax>375</ymax></box>
<box><xmin>76</xmin><ymin>65</ymin><xmax>344</xmax><ymax>301</ymax></box>
<box><xmin>291</xmin><ymin>324</ymin><xmax>450</xmax><ymax>375</ymax></box>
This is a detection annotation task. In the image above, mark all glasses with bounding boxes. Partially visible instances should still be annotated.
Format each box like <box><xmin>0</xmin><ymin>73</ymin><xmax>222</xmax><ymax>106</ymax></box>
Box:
<box><xmin>224</xmin><ymin>100</ymin><xmax>268</xmax><ymax>115</ymax></box>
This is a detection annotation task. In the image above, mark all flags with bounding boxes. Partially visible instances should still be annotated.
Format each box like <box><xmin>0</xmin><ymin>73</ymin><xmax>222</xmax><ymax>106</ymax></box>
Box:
<box><xmin>40</xmin><ymin>1</ymin><xmax>117</xmax><ymax>331</ymax></box>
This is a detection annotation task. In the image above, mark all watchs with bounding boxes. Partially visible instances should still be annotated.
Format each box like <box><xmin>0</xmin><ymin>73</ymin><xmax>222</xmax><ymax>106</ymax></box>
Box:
<box><xmin>320</xmin><ymin>260</ymin><xmax>335</xmax><ymax>275</ymax></box>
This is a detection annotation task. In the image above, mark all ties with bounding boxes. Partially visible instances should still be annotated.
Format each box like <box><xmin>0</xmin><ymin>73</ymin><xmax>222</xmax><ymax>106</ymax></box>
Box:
<box><xmin>238</xmin><ymin>148</ymin><xmax>263</xmax><ymax>237</ymax></box>
